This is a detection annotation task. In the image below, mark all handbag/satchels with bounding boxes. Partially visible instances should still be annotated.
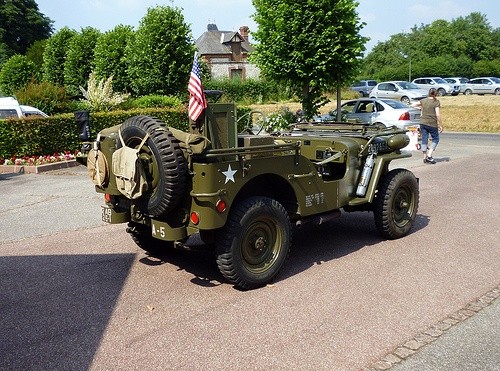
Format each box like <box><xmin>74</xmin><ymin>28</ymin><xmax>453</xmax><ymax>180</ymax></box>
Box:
<box><xmin>172</xmin><ymin>126</ymin><xmax>212</xmax><ymax>154</ymax></box>
<box><xmin>87</xmin><ymin>150</ymin><xmax>110</xmax><ymax>187</ymax></box>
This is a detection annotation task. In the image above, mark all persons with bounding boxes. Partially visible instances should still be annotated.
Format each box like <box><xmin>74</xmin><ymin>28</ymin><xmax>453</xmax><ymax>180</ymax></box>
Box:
<box><xmin>411</xmin><ymin>88</ymin><xmax>443</xmax><ymax>164</ymax></box>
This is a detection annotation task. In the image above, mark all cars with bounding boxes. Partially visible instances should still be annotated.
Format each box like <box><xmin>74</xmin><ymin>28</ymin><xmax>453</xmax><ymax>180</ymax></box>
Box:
<box><xmin>0</xmin><ymin>96</ymin><xmax>50</xmax><ymax>120</ymax></box>
<box><xmin>314</xmin><ymin>98</ymin><xmax>420</xmax><ymax>131</ymax></box>
<box><xmin>369</xmin><ymin>81</ymin><xmax>429</xmax><ymax>106</ymax></box>
<box><xmin>460</xmin><ymin>77</ymin><xmax>500</xmax><ymax>96</ymax></box>
<box><xmin>348</xmin><ymin>79</ymin><xmax>378</xmax><ymax>98</ymax></box>
<box><xmin>443</xmin><ymin>77</ymin><xmax>469</xmax><ymax>85</ymax></box>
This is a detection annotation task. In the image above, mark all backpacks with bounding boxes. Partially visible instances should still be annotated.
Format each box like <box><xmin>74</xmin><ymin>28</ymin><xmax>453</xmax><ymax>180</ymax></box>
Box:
<box><xmin>112</xmin><ymin>147</ymin><xmax>149</xmax><ymax>200</ymax></box>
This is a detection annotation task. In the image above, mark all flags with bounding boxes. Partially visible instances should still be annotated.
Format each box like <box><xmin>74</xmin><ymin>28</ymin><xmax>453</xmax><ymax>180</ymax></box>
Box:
<box><xmin>187</xmin><ymin>51</ymin><xmax>207</xmax><ymax>121</ymax></box>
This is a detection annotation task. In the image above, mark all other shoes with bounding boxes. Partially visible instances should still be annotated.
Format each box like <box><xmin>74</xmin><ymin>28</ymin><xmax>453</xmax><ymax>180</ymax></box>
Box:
<box><xmin>423</xmin><ymin>156</ymin><xmax>436</xmax><ymax>164</ymax></box>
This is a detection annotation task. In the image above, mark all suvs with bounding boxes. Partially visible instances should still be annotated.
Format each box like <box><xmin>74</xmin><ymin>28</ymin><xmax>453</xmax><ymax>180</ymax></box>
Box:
<box><xmin>412</xmin><ymin>77</ymin><xmax>461</xmax><ymax>96</ymax></box>
<box><xmin>87</xmin><ymin>50</ymin><xmax>420</xmax><ymax>290</ymax></box>
